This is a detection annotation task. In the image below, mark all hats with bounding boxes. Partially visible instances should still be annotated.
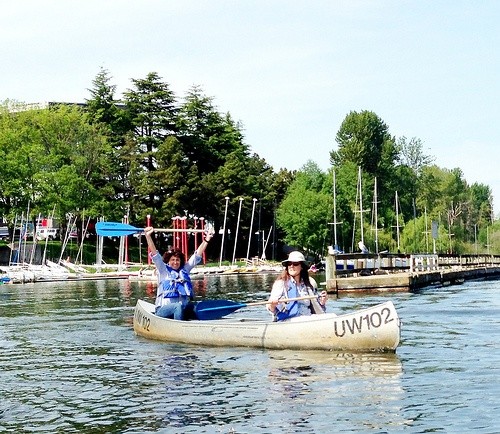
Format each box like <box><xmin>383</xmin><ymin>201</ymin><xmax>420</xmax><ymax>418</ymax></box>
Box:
<box><xmin>282</xmin><ymin>251</ymin><xmax>306</xmax><ymax>266</ymax></box>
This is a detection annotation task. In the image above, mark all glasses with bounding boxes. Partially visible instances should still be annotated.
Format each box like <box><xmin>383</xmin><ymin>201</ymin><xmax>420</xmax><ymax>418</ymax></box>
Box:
<box><xmin>286</xmin><ymin>262</ymin><xmax>302</xmax><ymax>267</ymax></box>
<box><xmin>165</xmin><ymin>250</ymin><xmax>182</xmax><ymax>256</ymax></box>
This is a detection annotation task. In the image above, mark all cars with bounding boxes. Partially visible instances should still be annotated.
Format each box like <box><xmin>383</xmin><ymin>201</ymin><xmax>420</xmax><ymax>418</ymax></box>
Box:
<box><xmin>70</xmin><ymin>229</ymin><xmax>90</xmax><ymax>239</ymax></box>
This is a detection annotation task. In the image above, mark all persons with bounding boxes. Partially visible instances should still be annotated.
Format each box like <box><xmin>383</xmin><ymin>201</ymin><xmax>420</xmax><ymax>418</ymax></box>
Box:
<box><xmin>144</xmin><ymin>226</ymin><xmax>215</xmax><ymax>320</ymax></box>
<box><xmin>266</xmin><ymin>251</ymin><xmax>328</xmax><ymax>322</ymax></box>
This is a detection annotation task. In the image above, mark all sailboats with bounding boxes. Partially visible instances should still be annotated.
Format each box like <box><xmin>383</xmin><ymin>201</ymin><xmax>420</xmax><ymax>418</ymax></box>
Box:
<box><xmin>0</xmin><ymin>196</ymin><xmax>286</xmax><ymax>284</ymax></box>
<box><xmin>321</xmin><ymin>164</ymin><xmax>500</xmax><ymax>278</ymax></box>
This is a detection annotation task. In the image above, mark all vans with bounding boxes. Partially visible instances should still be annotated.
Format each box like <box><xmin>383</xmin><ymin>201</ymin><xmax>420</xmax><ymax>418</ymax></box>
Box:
<box><xmin>36</xmin><ymin>229</ymin><xmax>60</xmax><ymax>241</ymax></box>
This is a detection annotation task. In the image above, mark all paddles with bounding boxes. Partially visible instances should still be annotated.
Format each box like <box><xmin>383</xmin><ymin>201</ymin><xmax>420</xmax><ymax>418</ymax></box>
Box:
<box><xmin>94</xmin><ymin>221</ymin><xmax>208</xmax><ymax>237</ymax></box>
<box><xmin>195</xmin><ymin>294</ymin><xmax>321</xmax><ymax>319</ymax></box>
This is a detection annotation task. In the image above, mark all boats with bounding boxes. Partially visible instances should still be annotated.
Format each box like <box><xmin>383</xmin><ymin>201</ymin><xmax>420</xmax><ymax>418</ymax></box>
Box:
<box><xmin>132</xmin><ymin>299</ymin><xmax>400</xmax><ymax>352</ymax></box>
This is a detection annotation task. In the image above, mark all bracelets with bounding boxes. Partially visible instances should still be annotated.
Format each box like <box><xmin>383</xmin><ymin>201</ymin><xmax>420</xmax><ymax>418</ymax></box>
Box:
<box><xmin>204</xmin><ymin>237</ymin><xmax>209</xmax><ymax>243</ymax></box>
